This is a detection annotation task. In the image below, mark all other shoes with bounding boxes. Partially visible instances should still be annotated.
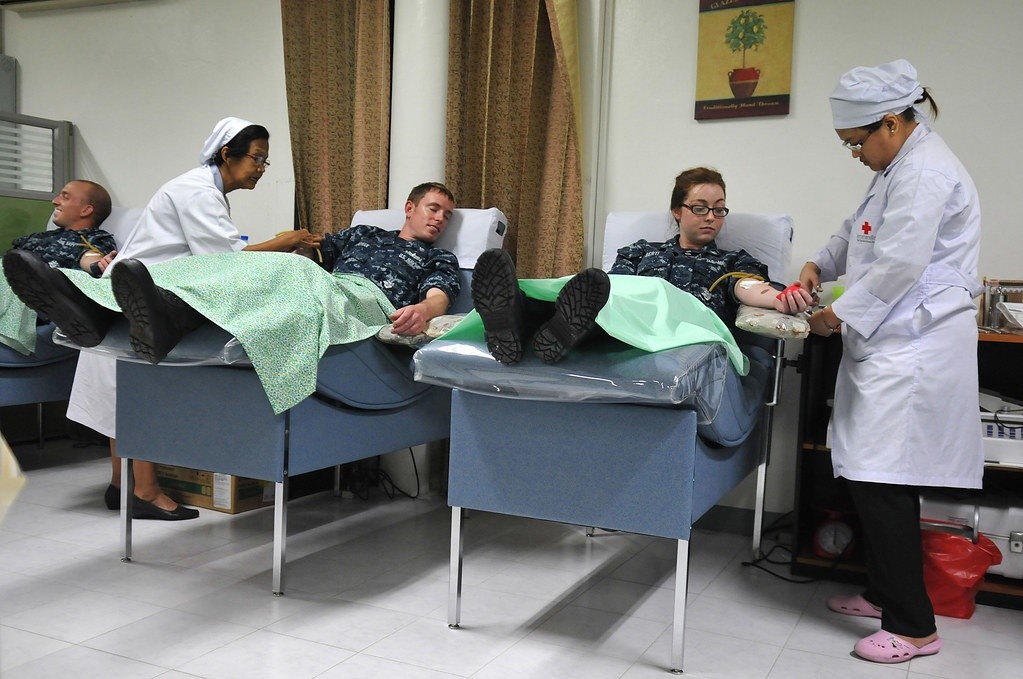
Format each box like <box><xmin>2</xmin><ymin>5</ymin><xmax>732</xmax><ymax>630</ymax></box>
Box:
<box><xmin>2</xmin><ymin>247</ymin><xmax>111</xmax><ymax>348</ymax></box>
<box><xmin>471</xmin><ymin>247</ymin><xmax>528</xmax><ymax>368</ymax></box>
<box><xmin>532</xmin><ymin>267</ymin><xmax>610</xmax><ymax>366</ymax></box>
<box><xmin>111</xmin><ymin>257</ymin><xmax>195</xmax><ymax>365</ymax></box>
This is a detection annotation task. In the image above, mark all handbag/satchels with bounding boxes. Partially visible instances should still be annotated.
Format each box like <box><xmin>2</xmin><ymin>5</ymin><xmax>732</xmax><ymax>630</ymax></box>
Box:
<box><xmin>920</xmin><ymin>519</ymin><xmax>1005</xmax><ymax>619</ymax></box>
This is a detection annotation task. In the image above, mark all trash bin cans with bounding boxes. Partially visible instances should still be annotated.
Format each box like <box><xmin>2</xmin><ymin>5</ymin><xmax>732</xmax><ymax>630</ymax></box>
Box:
<box><xmin>920</xmin><ymin>520</ymin><xmax>975</xmax><ymax>619</ymax></box>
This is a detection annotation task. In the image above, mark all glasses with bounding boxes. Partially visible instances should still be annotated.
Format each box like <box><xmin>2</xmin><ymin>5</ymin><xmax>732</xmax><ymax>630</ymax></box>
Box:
<box><xmin>842</xmin><ymin>127</ymin><xmax>876</xmax><ymax>153</ymax></box>
<box><xmin>681</xmin><ymin>202</ymin><xmax>730</xmax><ymax>218</ymax></box>
<box><xmin>245</xmin><ymin>153</ymin><xmax>271</xmax><ymax>168</ymax></box>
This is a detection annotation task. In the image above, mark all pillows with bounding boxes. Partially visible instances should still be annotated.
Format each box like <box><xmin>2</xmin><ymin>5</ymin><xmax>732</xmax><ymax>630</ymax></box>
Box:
<box><xmin>736</xmin><ymin>301</ymin><xmax>811</xmax><ymax>340</ymax></box>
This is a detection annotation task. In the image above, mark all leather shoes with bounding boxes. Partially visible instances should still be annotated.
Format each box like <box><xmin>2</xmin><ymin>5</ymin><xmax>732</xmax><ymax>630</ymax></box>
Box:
<box><xmin>130</xmin><ymin>493</ymin><xmax>200</xmax><ymax>521</ymax></box>
<box><xmin>104</xmin><ymin>484</ymin><xmax>132</xmax><ymax>510</ymax></box>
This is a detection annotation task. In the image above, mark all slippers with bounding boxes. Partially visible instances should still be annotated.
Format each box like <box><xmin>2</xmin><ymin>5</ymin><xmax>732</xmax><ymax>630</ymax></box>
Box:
<box><xmin>854</xmin><ymin>629</ymin><xmax>942</xmax><ymax>664</ymax></box>
<box><xmin>826</xmin><ymin>593</ymin><xmax>882</xmax><ymax>618</ymax></box>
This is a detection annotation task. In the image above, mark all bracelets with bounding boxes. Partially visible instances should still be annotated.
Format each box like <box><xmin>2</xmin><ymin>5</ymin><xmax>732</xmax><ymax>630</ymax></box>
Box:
<box><xmin>821</xmin><ymin>310</ymin><xmax>840</xmax><ymax>332</ymax></box>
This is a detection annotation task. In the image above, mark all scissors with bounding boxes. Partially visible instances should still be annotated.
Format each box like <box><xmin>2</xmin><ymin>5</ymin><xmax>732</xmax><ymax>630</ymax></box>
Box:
<box><xmin>811</xmin><ymin>284</ymin><xmax>821</xmax><ymax>302</ymax></box>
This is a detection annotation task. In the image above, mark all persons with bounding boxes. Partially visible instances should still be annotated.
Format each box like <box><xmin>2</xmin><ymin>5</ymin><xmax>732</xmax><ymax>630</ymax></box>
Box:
<box><xmin>2</xmin><ymin>181</ymin><xmax>462</xmax><ymax>364</ymax></box>
<box><xmin>10</xmin><ymin>179</ymin><xmax>118</xmax><ymax>279</ymax></box>
<box><xmin>471</xmin><ymin>166</ymin><xmax>814</xmax><ymax>367</ymax></box>
<box><xmin>798</xmin><ymin>58</ymin><xmax>984</xmax><ymax>663</ymax></box>
<box><xmin>66</xmin><ymin>117</ymin><xmax>323</xmax><ymax>519</ymax></box>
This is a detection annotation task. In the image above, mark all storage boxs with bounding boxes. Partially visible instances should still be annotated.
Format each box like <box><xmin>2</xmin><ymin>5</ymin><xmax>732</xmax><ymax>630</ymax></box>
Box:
<box><xmin>912</xmin><ymin>489</ymin><xmax>1023</xmax><ymax>580</ymax></box>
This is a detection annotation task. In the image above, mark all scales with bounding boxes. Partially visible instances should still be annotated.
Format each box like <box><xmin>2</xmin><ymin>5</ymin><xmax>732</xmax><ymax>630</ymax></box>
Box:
<box><xmin>811</xmin><ymin>493</ymin><xmax>858</xmax><ymax>562</ymax></box>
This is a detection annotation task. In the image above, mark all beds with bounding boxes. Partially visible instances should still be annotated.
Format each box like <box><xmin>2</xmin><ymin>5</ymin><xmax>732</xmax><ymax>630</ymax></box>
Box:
<box><xmin>1</xmin><ymin>203</ymin><xmax>149</xmax><ymax>447</ymax></box>
<box><xmin>412</xmin><ymin>216</ymin><xmax>794</xmax><ymax>676</ymax></box>
<box><xmin>102</xmin><ymin>204</ymin><xmax>510</xmax><ymax>595</ymax></box>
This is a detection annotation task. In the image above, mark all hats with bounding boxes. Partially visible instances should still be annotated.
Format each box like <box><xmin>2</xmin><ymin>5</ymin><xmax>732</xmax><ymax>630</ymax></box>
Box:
<box><xmin>198</xmin><ymin>116</ymin><xmax>257</xmax><ymax>166</ymax></box>
<box><xmin>829</xmin><ymin>57</ymin><xmax>924</xmax><ymax>130</ymax></box>
